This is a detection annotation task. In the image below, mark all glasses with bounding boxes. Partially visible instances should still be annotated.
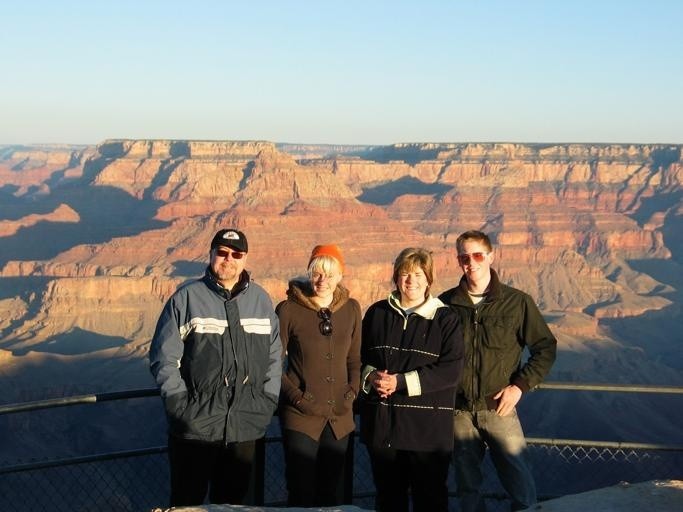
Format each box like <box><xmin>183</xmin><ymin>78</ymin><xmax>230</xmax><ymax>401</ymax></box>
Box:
<box><xmin>216</xmin><ymin>250</ymin><xmax>243</xmax><ymax>259</ymax></box>
<box><xmin>319</xmin><ymin>308</ymin><xmax>333</xmax><ymax>336</ymax></box>
<box><xmin>457</xmin><ymin>253</ymin><xmax>484</xmax><ymax>265</ymax></box>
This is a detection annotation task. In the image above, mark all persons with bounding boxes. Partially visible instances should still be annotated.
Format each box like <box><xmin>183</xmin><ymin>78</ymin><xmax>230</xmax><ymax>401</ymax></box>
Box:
<box><xmin>274</xmin><ymin>244</ymin><xmax>362</xmax><ymax>507</ymax></box>
<box><xmin>149</xmin><ymin>229</ymin><xmax>282</xmax><ymax>507</ymax></box>
<box><xmin>360</xmin><ymin>248</ymin><xmax>464</xmax><ymax>512</ymax></box>
<box><xmin>438</xmin><ymin>230</ymin><xmax>557</xmax><ymax>512</ymax></box>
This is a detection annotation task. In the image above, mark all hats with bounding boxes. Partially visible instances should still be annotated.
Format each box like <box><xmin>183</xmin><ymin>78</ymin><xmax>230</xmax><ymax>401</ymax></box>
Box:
<box><xmin>307</xmin><ymin>244</ymin><xmax>346</xmax><ymax>274</ymax></box>
<box><xmin>211</xmin><ymin>229</ymin><xmax>248</xmax><ymax>252</ymax></box>
